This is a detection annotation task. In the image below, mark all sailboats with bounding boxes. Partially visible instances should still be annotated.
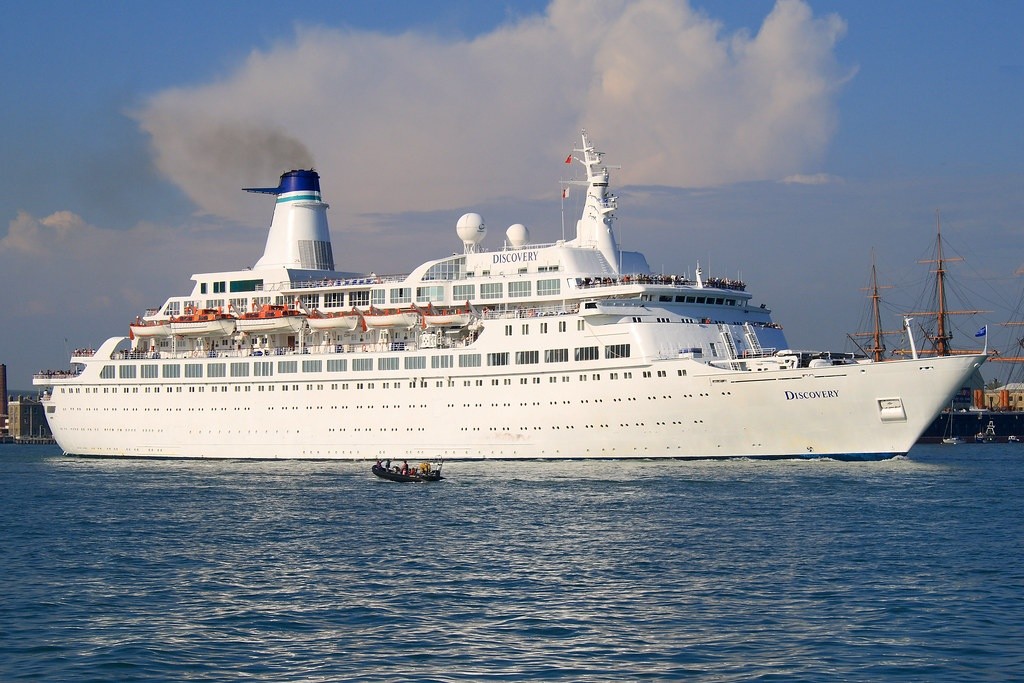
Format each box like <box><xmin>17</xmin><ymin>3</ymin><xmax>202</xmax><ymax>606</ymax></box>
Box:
<box><xmin>846</xmin><ymin>218</ymin><xmax>1024</xmax><ymax>437</ymax></box>
<box><xmin>943</xmin><ymin>400</ymin><xmax>967</xmax><ymax>443</ymax></box>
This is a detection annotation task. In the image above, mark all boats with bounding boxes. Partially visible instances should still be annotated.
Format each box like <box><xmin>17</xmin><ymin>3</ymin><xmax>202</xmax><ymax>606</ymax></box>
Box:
<box><xmin>976</xmin><ymin>417</ymin><xmax>1010</xmax><ymax>443</ymax></box>
<box><xmin>169</xmin><ymin>303</ymin><xmax>237</xmax><ymax>337</ymax></box>
<box><xmin>229</xmin><ymin>298</ymin><xmax>303</xmax><ymax>335</ymax></box>
<box><xmin>352</xmin><ymin>304</ymin><xmax>420</xmax><ymax>332</ymax></box>
<box><xmin>32</xmin><ymin>129</ymin><xmax>994</xmax><ymax>462</ymax></box>
<box><xmin>411</xmin><ymin>301</ymin><xmax>471</xmax><ymax>329</ymax></box>
<box><xmin>129</xmin><ymin>315</ymin><xmax>171</xmax><ymax>336</ymax></box>
<box><xmin>372</xmin><ymin>465</ymin><xmax>441</xmax><ymax>482</ymax></box>
<box><xmin>297</xmin><ymin>296</ymin><xmax>358</xmax><ymax>334</ymax></box>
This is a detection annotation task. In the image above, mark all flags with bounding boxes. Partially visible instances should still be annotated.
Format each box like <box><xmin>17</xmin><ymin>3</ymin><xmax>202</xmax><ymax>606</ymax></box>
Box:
<box><xmin>563</xmin><ymin>187</ymin><xmax>569</xmax><ymax>198</ymax></box>
<box><xmin>975</xmin><ymin>327</ymin><xmax>985</xmax><ymax>337</ymax></box>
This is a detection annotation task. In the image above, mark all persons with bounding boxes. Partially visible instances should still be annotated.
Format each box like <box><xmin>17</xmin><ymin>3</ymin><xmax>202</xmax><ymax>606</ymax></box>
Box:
<box><xmin>377</xmin><ymin>460</ymin><xmax>431</xmax><ymax>476</ymax></box>
<box><xmin>482</xmin><ymin>306</ymin><xmax>491</xmax><ymax>320</ymax></box>
<box><xmin>369</xmin><ymin>272</ymin><xmax>376</xmax><ymax>284</ymax></box>
<box><xmin>119</xmin><ymin>345</ymin><xmax>149</xmax><ymax>359</ymax></box>
<box><xmin>706</xmin><ymin>318</ymin><xmax>710</xmax><ymax>323</ymax></box>
<box><xmin>75</xmin><ymin>347</ymin><xmax>96</xmax><ymax>357</ymax></box>
<box><xmin>705</xmin><ymin>278</ymin><xmax>746</xmax><ymax>291</ymax></box>
<box><xmin>588</xmin><ymin>273</ymin><xmax>692</xmax><ymax>288</ymax></box>
<box><xmin>753</xmin><ymin>320</ymin><xmax>783</xmax><ymax>330</ymax></box>
<box><xmin>39</xmin><ymin>369</ymin><xmax>72</xmax><ymax>379</ymax></box>
